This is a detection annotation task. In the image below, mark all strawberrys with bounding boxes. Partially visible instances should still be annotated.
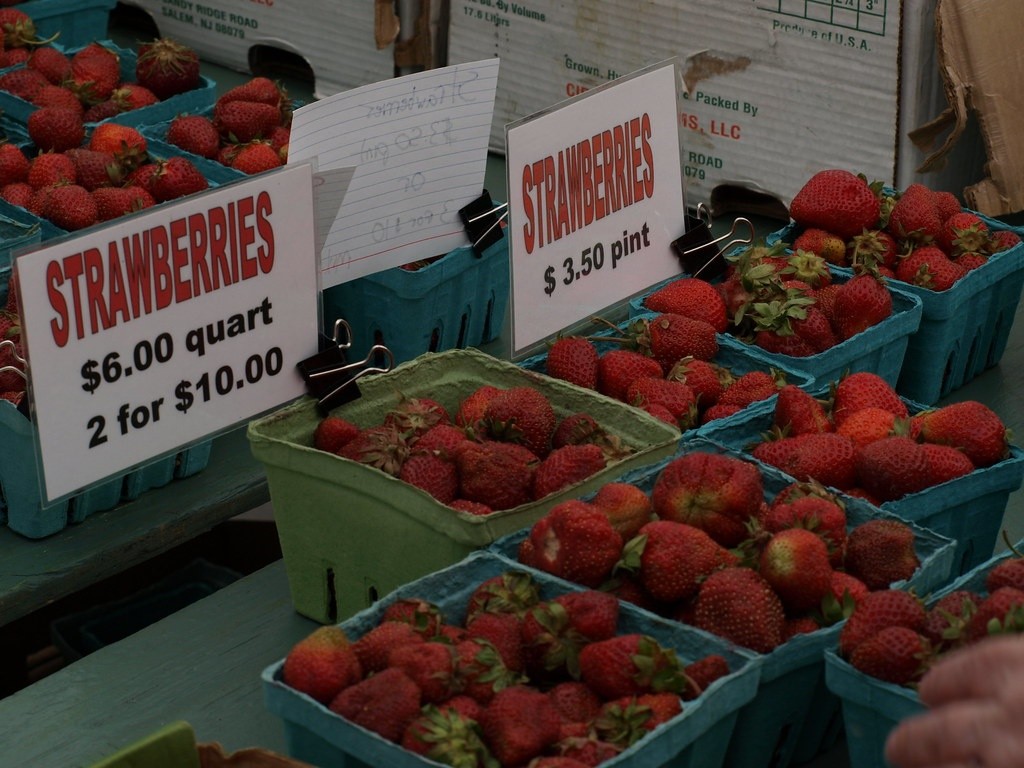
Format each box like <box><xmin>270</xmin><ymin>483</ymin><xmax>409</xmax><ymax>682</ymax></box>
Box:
<box><xmin>0</xmin><ymin>7</ymin><xmax>1023</xmax><ymax>767</ymax></box>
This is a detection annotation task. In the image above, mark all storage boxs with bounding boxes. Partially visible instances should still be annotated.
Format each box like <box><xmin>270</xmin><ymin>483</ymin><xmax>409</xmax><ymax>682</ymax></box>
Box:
<box><xmin>246</xmin><ymin>347</ymin><xmax>681</xmax><ymax>627</ymax></box>
<box><xmin>1</xmin><ymin>121</ymin><xmax>219</xmax><ymax>242</ymax></box>
<box><xmin>0</xmin><ymin>197</ymin><xmax>41</xmax><ymax>306</ymax></box>
<box><xmin>767</xmin><ymin>189</ymin><xmax>1024</xmax><ymax>406</ymax></box>
<box><xmin>135</xmin><ymin>103</ymin><xmax>304</xmax><ymax>185</ymax></box>
<box><xmin>324</xmin><ymin>199</ymin><xmax>511</xmax><ymax>373</ymax></box>
<box><xmin>91</xmin><ymin>720</ymin><xmax>319</xmax><ymax>767</ymax></box>
<box><xmin>514</xmin><ymin>313</ymin><xmax>816</xmax><ymax>434</ymax></box>
<box><xmin>703</xmin><ymin>377</ymin><xmax>1024</xmax><ymax>584</ymax></box>
<box><xmin>496</xmin><ymin>438</ymin><xmax>957</xmax><ymax>768</ymax></box>
<box><xmin>1</xmin><ymin>397</ymin><xmax>214</xmax><ymax>538</ymax></box>
<box><xmin>439</xmin><ymin>2</ymin><xmax>1024</xmax><ymax>226</ymax></box>
<box><xmin>263</xmin><ymin>549</ymin><xmax>760</xmax><ymax>768</ymax></box>
<box><xmin>0</xmin><ymin>35</ymin><xmax>67</xmax><ymax>71</ymax></box>
<box><xmin>130</xmin><ymin>2</ymin><xmax>438</xmax><ymax>92</ymax></box>
<box><xmin>629</xmin><ymin>247</ymin><xmax>922</xmax><ymax>398</ymax></box>
<box><xmin>0</xmin><ymin>37</ymin><xmax>216</xmax><ymax>138</ymax></box>
<box><xmin>825</xmin><ymin>542</ymin><xmax>1023</xmax><ymax>767</ymax></box>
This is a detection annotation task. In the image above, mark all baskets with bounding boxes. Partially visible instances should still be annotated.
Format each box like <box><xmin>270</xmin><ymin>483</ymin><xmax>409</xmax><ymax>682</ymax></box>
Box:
<box><xmin>0</xmin><ymin>0</ymin><xmax>1024</xmax><ymax>768</ymax></box>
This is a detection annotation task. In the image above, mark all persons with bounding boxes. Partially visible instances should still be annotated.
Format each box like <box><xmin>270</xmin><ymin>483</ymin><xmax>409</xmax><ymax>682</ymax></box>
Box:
<box><xmin>883</xmin><ymin>628</ymin><xmax>1024</xmax><ymax>766</ymax></box>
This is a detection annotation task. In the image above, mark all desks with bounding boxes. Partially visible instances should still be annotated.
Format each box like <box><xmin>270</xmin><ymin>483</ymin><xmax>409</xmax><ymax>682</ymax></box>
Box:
<box><xmin>3</xmin><ymin>430</ymin><xmax>269</xmax><ymax>617</ymax></box>
<box><xmin>0</xmin><ymin>560</ymin><xmax>364</xmax><ymax>767</ymax></box>
